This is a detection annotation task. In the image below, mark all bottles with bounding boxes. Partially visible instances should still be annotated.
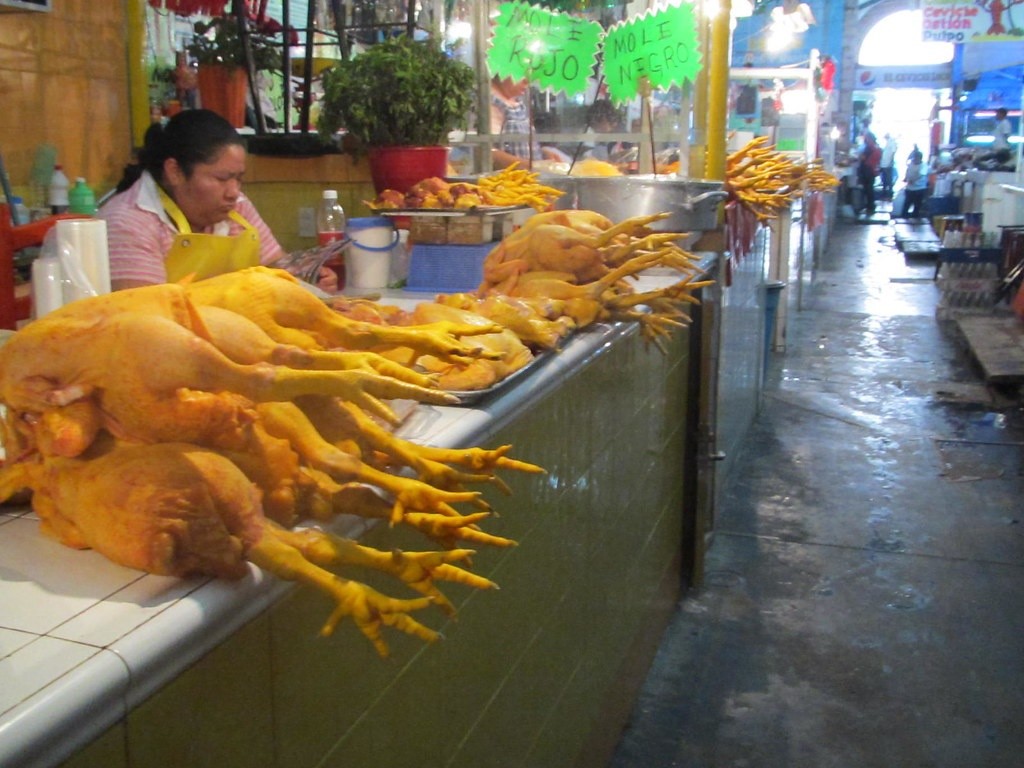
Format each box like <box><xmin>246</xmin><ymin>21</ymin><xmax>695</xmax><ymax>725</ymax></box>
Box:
<box><xmin>167</xmin><ymin>101</ymin><xmax>180</xmax><ymax>117</ymax></box>
<box><xmin>150</xmin><ymin>99</ymin><xmax>162</xmax><ymax>124</ymax></box>
<box><xmin>317</xmin><ymin>189</ymin><xmax>346</xmax><ymax>290</ymax></box>
<box><xmin>47</xmin><ymin>166</ymin><xmax>68</xmax><ymax>205</ymax></box>
<box><xmin>11</xmin><ymin>196</ymin><xmax>30</xmax><ymax>227</ymax></box>
<box><xmin>68</xmin><ymin>177</ymin><xmax>96</xmax><ymax>214</ymax></box>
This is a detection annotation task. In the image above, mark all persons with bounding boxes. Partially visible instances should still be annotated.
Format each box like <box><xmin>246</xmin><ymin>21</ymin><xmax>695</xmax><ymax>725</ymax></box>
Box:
<box><xmin>859</xmin><ymin>132</ymin><xmax>881</xmax><ymax>214</ymax></box>
<box><xmin>973</xmin><ymin>148</ymin><xmax>1015</xmax><ymax>172</ymax></box>
<box><xmin>880</xmin><ymin>133</ymin><xmax>897</xmax><ymax>190</ymax></box>
<box><xmin>587</xmin><ymin>99</ymin><xmax>632</xmax><ymax>159</ymax></box>
<box><xmin>95</xmin><ymin>109</ymin><xmax>337</xmax><ymax>293</ymax></box>
<box><xmin>467</xmin><ymin>72</ymin><xmax>562</xmax><ymax>170</ymax></box>
<box><xmin>903</xmin><ymin>143</ymin><xmax>927</xmax><ymax>217</ymax></box>
<box><xmin>992</xmin><ymin>109</ymin><xmax>1011</xmax><ymax>149</ymax></box>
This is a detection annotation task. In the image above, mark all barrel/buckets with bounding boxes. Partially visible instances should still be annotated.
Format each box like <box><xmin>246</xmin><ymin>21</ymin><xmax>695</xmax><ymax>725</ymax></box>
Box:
<box><xmin>346</xmin><ymin>216</ymin><xmax>399</xmax><ymax>288</ymax></box>
<box><xmin>764</xmin><ymin>278</ymin><xmax>787</xmax><ymax>386</ymax></box>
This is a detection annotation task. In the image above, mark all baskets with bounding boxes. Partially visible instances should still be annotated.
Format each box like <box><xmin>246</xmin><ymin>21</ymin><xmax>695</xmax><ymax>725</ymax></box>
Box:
<box><xmin>402</xmin><ymin>242</ymin><xmax>499</xmax><ymax>293</ymax></box>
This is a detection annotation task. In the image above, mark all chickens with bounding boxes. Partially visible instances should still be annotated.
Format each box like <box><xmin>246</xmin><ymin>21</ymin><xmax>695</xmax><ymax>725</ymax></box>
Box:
<box><xmin>1</xmin><ymin>131</ymin><xmax>842</xmax><ymax>661</ymax></box>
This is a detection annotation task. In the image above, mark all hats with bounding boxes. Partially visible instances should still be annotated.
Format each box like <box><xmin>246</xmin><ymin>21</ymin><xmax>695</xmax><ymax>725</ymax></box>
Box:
<box><xmin>994</xmin><ymin>108</ymin><xmax>1007</xmax><ymax>116</ymax></box>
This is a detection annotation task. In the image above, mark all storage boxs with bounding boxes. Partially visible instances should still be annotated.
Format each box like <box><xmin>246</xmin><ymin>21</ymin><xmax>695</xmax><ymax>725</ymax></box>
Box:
<box><xmin>402</xmin><ymin>208</ymin><xmax>536</xmax><ymax>292</ymax></box>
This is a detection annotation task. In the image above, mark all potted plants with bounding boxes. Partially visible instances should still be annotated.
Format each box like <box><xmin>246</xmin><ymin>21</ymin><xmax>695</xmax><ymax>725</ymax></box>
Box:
<box><xmin>316</xmin><ymin>35</ymin><xmax>480</xmax><ymax>234</ymax></box>
<box><xmin>182</xmin><ymin>15</ymin><xmax>283</xmax><ymax>129</ymax></box>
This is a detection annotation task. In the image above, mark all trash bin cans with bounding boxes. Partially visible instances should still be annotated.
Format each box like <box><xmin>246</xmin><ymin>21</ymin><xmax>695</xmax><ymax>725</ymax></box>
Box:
<box><xmin>764</xmin><ymin>279</ymin><xmax>786</xmax><ymax>379</ymax></box>
